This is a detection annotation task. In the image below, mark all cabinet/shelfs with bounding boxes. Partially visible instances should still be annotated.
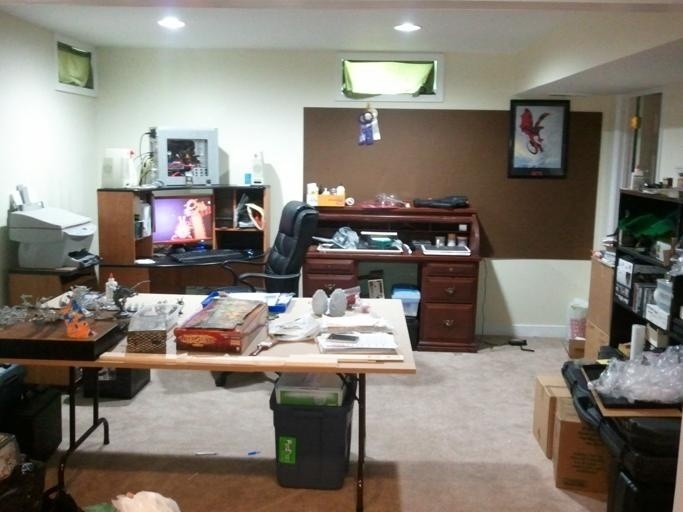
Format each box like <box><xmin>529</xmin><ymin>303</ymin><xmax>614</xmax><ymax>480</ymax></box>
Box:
<box><xmin>3</xmin><ymin>263</ymin><xmax>99</xmax><ymax>393</ymax></box>
<box><xmin>610</xmin><ymin>188</ymin><xmax>683</xmax><ymax>356</ymax></box>
<box><xmin>303</xmin><ymin>204</ymin><xmax>482</xmax><ymax>352</ymax></box>
<box><xmin>96</xmin><ymin>184</ymin><xmax>271</xmax><ymax>265</ymax></box>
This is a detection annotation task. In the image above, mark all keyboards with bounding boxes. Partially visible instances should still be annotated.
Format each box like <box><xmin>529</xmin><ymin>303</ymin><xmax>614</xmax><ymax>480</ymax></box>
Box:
<box><xmin>169</xmin><ymin>248</ymin><xmax>241</xmax><ymax>263</ymax></box>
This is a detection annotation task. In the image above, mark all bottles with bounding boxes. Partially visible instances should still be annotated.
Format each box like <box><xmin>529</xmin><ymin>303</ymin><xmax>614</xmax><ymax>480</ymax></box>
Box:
<box><xmin>105</xmin><ymin>271</ymin><xmax>118</xmax><ymax>303</ymax></box>
<box><xmin>456</xmin><ymin>224</ymin><xmax>469</xmax><ymax>247</ymax></box>
<box><xmin>631</xmin><ymin>165</ymin><xmax>644</xmax><ymax>191</ymax></box>
<box><xmin>329</xmin><ymin>287</ymin><xmax>346</xmax><ymax>317</ymax></box>
<box><xmin>312</xmin><ymin>287</ymin><xmax>328</xmax><ymax>316</ymax></box>
<box><xmin>447</xmin><ymin>234</ymin><xmax>456</xmax><ymax>246</ymax></box>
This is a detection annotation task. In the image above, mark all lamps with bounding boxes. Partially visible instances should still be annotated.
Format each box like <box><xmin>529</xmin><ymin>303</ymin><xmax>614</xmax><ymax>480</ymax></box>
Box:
<box><xmin>392</xmin><ymin>19</ymin><xmax>423</xmax><ymax>33</ymax></box>
<box><xmin>155</xmin><ymin>15</ymin><xmax>186</xmax><ymax>31</ymax></box>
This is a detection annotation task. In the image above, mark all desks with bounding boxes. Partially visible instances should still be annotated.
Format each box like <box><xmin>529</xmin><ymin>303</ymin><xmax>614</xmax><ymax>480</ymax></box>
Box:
<box><xmin>1</xmin><ymin>289</ymin><xmax>417</xmax><ymax>511</ymax></box>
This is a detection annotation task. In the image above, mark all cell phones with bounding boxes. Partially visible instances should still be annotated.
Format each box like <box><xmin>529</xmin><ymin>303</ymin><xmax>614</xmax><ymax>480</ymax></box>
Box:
<box><xmin>326</xmin><ymin>333</ymin><xmax>359</xmax><ymax>344</ymax></box>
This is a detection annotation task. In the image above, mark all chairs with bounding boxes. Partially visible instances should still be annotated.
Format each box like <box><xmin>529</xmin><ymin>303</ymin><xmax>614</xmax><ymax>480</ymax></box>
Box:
<box><xmin>173</xmin><ymin>200</ymin><xmax>319</xmax><ymax>297</ymax></box>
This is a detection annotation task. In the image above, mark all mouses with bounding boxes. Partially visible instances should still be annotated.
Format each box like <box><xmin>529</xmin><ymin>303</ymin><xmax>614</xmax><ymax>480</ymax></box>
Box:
<box><xmin>242</xmin><ymin>248</ymin><xmax>255</xmax><ymax>256</ymax></box>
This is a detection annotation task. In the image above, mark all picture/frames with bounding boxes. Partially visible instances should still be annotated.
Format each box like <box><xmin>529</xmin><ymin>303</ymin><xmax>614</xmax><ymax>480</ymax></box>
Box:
<box><xmin>54</xmin><ymin>33</ymin><xmax>98</xmax><ymax>99</ymax></box>
<box><xmin>335</xmin><ymin>51</ymin><xmax>445</xmax><ymax>103</ymax></box>
<box><xmin>156</xmin><ymin>126</ymin><xmax>220</xmax><ymax>186</ymax></box>
<box><xmin>507</xmin><ymin>98</ymin><xmax>571</xmax><ymax>180</ymax></box>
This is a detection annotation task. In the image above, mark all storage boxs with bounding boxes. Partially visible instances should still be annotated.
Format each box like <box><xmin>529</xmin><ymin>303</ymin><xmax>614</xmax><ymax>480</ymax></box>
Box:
<box><xmin>551</xmin><ymin>396</ymin><xmax>612</xmax><ymax>503</ymax></box>
<box><xmin>581</xmin><ymin>319</ymin><xmax>610</xmax><ymax>362</ymax></box>
<box><xmin>586</xmin><ymin>257</ymin><xmax>616</xmax><ymax>338</ymax></box>
<box><xmin>531</xmin><ymin>373</ymin><xmax>572</xmax><ymax>460</ymax></box>
<box><xmin>568</xmin><ymin>338</ymin><xmax>585</xmax><ymax>360</ymax></box>
<box><xmin>80</xmin><ymin>363</ymin><xmax>151</xmax><ymax>399</ymax></box>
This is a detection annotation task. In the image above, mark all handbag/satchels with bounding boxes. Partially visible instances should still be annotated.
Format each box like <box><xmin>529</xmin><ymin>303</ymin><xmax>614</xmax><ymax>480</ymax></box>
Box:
<box><xmin>413</xmin><ymin>195</ymin><xmax>471</xmax><ymax>209</ymax></box>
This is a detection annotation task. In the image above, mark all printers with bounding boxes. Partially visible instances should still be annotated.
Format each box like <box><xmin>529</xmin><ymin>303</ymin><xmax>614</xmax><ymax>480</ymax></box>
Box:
<box><xmin>7</xmin><ymin>182</ymin><xmax>103</xmax><ymax>275</ymax></box>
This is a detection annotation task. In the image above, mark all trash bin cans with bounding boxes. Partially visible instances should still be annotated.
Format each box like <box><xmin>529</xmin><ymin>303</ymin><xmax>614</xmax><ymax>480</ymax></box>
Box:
<box><xmin>571</xmin><ymin>308</ymin><xmax>588</xmax><ymax>339</ymax></box>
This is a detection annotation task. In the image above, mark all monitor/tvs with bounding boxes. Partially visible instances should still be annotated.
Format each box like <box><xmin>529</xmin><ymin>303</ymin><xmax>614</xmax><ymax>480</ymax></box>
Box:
<box><xmin>151</xmin><ymin>188</ymin><xmax>213</xmax><ymax>248</ymax></box>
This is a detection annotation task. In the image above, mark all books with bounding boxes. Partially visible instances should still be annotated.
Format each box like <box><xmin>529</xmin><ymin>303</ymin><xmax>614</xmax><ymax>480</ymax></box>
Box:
<box><xmin>218</xmin><ymin>288</ymin><xmax>404</xmax><ymax>364</ymax></box>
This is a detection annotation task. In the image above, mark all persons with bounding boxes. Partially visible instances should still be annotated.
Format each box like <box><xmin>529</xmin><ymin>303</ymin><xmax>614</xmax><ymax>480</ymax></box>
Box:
<box><xmin>172</xmin><ymin>199</ymin><xmax>205</xmax><ymax>239</ymax></box>
